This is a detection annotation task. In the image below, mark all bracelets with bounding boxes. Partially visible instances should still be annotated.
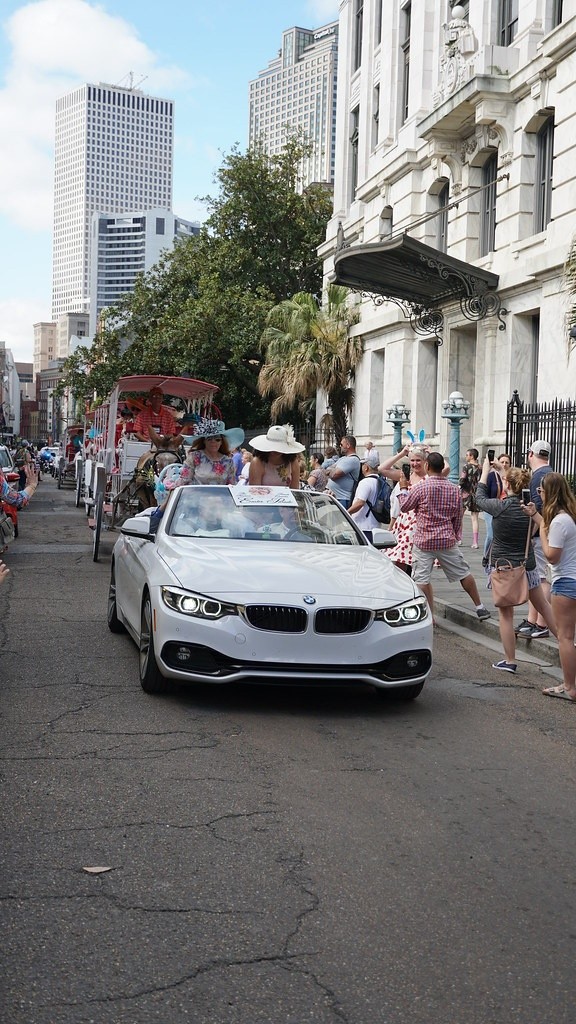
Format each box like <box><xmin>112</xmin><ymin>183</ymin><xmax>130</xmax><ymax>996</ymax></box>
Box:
<box><xmin>27</xmin><ymin>484</ymin><xmax>36</xmax><ymax>491</ymax></box>
<box><xmin>531</xmin><ymin>511</ymin><xmax>537</xmax><ymax>517</ymax></box>
<box><xmin>400</xmin><ymin>486</ymin><xmax>408</xmax><ymax>490</ymax></box>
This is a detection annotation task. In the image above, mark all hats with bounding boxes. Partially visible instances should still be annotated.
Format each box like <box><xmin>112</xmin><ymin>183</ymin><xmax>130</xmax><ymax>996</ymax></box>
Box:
<box><xmin>177</xmin><ymin>413</ymin><xmax>200</xmax><ymax>424</ymax></box>
<box><xmin>526</xmin><ymin>441</ymin><xmax>551</xmax><ymax>458</ymax></box>
<box><xmin>182</xmin><ymin>418</ymin><xmax>245</xmax><ymax>451</ymax></box>
<box><xmin>249</xmin><ymin>422</ymin><xmax>305</xmax><ymax>454</ymax></box>
<box><xmin>149</xmin><ymin>387</ymin><xmax>163</xmax><ymax>396</ymax></box>
<box><xmin>126</xmin><ymin>395</ymin><xmax>151</xmax><ymax>412</ymax></box>
<box><xmin>360</xmin><ymin>455</ymin><xmax>380</xmax><ymax>469</ymax></box>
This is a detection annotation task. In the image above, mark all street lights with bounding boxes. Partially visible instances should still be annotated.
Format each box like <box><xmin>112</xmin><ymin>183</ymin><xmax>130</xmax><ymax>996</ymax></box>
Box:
<box><xmin>439</xmin><ymin>389</ymin><xmax>471</xmax><ymax>486</ymax></box>
<box><xmin>385</xmin><ymin>400</ymin><xmax>411</xmax><ymax>486</ymax></box>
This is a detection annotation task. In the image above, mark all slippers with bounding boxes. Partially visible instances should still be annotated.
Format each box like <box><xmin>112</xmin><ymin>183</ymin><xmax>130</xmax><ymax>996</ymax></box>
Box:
<box><xmin>541</xmin><ymin>684</ymin><xmax>576</xmax><ymax>702</ymax></box>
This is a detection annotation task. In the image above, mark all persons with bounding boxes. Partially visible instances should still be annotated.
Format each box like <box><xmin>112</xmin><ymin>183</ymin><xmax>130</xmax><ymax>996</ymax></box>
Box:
<box><xmin>295</xmin><ymin>452</ymin><xmax>306</xmax><ymax>479</ymax></box>
<box><xmin>396</xmin><ymin>452</ymin><xmax>492</xmax><ymax>628</ymax></box>
<box><xmin>512</xmin><ymin>440</ymin><xmax>554</xmax><ymax>639</ymax></box>
<box><xmin>520</xmin><ymin>472</ymin><xmax>576</xmax><ymax>701</ymax></box>
<box><xmin>10</xmin><ymin>439</ymin><xmax>43</xmax><ymax>491</ymax></box>
<box><xmin>459</xmin><ymin>448</ymin><xmax>484</xmax><ymax>549</ymax></box>
<box><xmin>230</xmin><ymin>445</ymin><xmax>253</xmax><ymax>485</ymax></box>
<box><xmin>273</xmin><ymin>492</ymin><xmax>307</xmax><ymax>540</ymax></box>
<box><xmin>474</xmin><ymin>454</ymin><xmax>558</xmax><ymax>673</ymax></box>
<box><xmin>248</xmin><ymin>425</ymin><xmax>306</xmax><ymax>488</ymax></box>
<box><xmin>323</xmin><ymin>435</ymin><xmax>362</xmax><ymax>508</ymax></box>
<box><xmin>361</xmin><ymin>441</ymin><xmax>379</xmax><ymax>462</ymax></box>
<box><xmin>0</xmin><ymin>462</ymin><xmax>41</xmax><ymax>583</ymax></box>
<box><xmin>117</xmin><ymin>387</ymin><xmax>175</xmax><ymax>442</ymax></box>
<box><xmin>483</xmin><ymin>454</ymin><xmax>513</xmax><ymax>555</ymax></box>
<box><xmin>307</xmin><ymin>452</ymin><xmax>326</xmax><ymax>492</ymax></box>
<box><xmin>73</xmin><ymin>430</ymin><xmax>84</xmax><ymax>453</ymax></box>
<box><xmin>149</xmin><ymin>487</ymin><xmax>244</xmax><ymax>538</ymax></box>
<box><xmin>378</xmin><ymin>442</ymin><xmax>450</xmax><ymax>574</ymax></box>
<box><xmin>389</xmin><ymin>448</ymin><xmax>410</xmax><ymax>532</ymax></box>
<box><xmin>345</xmin><ymin>457</ymin><xmax>382</xmax><ymax>547</ymax></box>
<box><xmin>173</xmin><ymin>415</ymin><xmax>245</xmax><ymax>485</ymax></box>
<box><xmin>322</xmin><ymin>446</ymin><xmax>340</xmax><ymax>469</ymax></box>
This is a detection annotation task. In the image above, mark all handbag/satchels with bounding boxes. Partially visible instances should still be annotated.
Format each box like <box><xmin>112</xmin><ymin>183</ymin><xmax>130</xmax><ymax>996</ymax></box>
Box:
<box><xmin>0</xmin><ymin>510</ymin><xmax>15</xmax><ymax>550</ymax></box>
<box><xmin>490</xmin><ymin>558</ymin><xmax>530</xmax><ymax>608</ymax></box>
<box><xmin>457</xmin><ymin>484</ymin><xmax>470</xmax><ymax>507</ymax></box>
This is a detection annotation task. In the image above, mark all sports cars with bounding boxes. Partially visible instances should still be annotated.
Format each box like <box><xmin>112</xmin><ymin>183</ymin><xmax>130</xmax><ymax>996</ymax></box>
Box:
<box><xmin>113</xmin><ymin>483</ymin><xmax>434</xmax><ymax>702</ymax></box>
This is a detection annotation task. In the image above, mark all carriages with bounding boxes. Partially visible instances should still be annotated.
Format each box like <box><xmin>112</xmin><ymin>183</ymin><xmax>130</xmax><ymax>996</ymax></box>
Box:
<box><xmin>53</xmin><ymin>374</ymin><xmax>222</xmax><ymax>563</ymax></box>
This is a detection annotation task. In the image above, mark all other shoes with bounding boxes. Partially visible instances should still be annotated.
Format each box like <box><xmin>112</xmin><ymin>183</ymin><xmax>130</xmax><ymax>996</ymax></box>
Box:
<box><xmin>457</xmin><ymin>540</ymin><xmax>462</xmax><ymax>545</ymax></box>
<box><xmin>482</xmin><ymin>557</ymin><xmax>489</xmax><ymax>567</ymax></box>
<box><xmin>471</xmin><ymin>544</ymin><xmax>479</xmax><ymax>548</ymax></box>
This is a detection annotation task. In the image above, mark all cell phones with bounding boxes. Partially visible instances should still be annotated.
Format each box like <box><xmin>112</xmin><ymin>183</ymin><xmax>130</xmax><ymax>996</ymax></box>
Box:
<box><xmin>487</xmin><ymin>449</ymin><xmax>495</xmax><ymax>465</ymax></box>
<box><xmin>401</xmin><ymin>464</ymin><xmax>410</xmax><ymax>481</ymax></box>
<box><xmin>522</xmin><ymin>489</ymin><xmax>531</xmax><ymax>506</ymax></box>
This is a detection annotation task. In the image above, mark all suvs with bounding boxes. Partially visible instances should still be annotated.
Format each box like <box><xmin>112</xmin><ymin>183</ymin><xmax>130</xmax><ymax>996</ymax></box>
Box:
<box><xmin>0</xmin><ymin>444</ymin><xmax>21</xmax><ymax>495</ymax></box>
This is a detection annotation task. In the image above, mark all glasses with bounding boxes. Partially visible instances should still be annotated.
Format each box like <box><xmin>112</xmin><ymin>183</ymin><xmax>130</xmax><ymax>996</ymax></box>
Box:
<box><xmin>340</xmin><ymin>443</ymin><xmax>343</xmax><ymax>447</ymax></box>
<box><xmin>131</xmin><ymin>406</ymin><xmax>141</xmax><ymax>413</ymax></box>
<box><xmin>204</xmin><ymin>437</ymin><xmax>222</xmax><ymax>442</ymax></box>
<box><xmin>536</xmin><ymin>487</ymin><xmax>545</xmax><ymax>495</ymax></box>
<box><xmin>502</xmin><ymin>476</ymin><xmax>511</xmax><ymax>482</ymax></box>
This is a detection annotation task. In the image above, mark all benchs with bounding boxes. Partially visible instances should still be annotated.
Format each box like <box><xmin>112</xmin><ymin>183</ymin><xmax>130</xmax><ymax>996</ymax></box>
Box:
<box><xmin>65</xmin><ymin>422</ymin><xmax>192</xmax><ymax>475</ymax></box>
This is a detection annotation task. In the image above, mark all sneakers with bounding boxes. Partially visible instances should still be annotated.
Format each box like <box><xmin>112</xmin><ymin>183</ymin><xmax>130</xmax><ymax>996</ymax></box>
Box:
<box><xmin>516</xmin><ymin>623</ymin><xmax>549</xmax><ymax>638</ymax></box>
<box><xmin>492</xmin><ymin>660</ymin><xmax>518</xmax><ymax>673</ymax></box>
<box><xmin>476</xmin><ymin>608</ymin><xmax>491</xmax><ymax>620</ymax></box>
<box><xmin>515</xmin><ymin>618</ymin><xmax>534</xmax><ymax>634</ymax></box>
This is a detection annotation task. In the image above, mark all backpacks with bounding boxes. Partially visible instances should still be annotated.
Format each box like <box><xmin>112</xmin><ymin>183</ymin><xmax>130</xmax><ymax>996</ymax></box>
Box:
<box><xmin>362</xmin><ymin>475</ymin><xmax>392</xmax><ymax>524</ymax></box>
<box><xmin>348</xmin><ymin>455</ymin><xmax>365</xmax><ymax>507</ymax></box>
